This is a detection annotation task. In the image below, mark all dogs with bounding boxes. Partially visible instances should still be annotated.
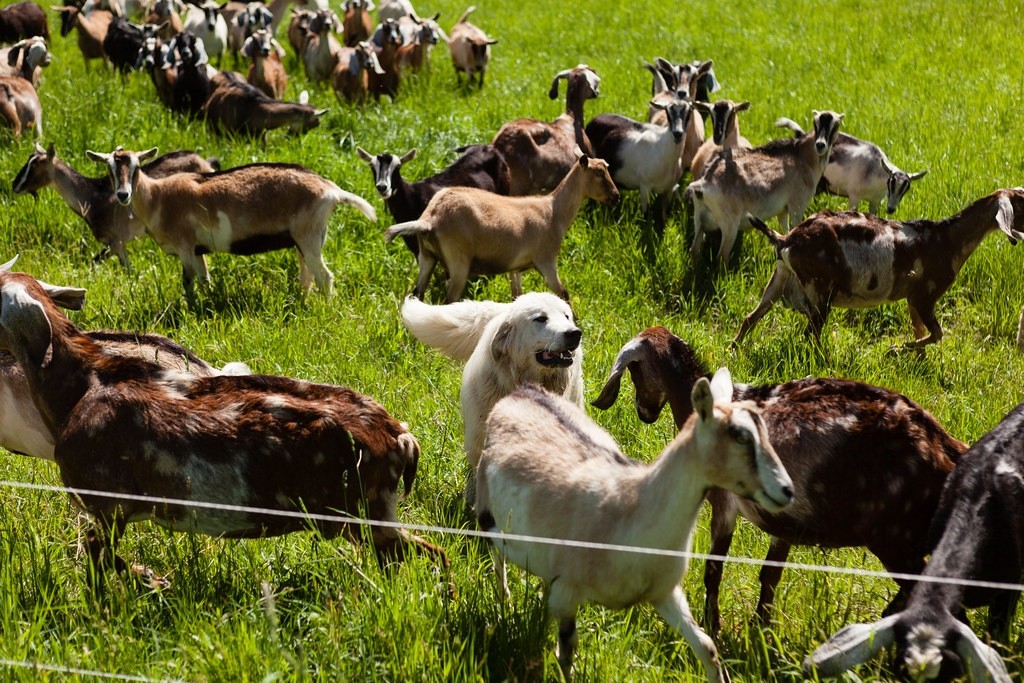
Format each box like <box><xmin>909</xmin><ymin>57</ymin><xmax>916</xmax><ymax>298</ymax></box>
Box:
<box><xmin>397</xmin><ymin>288</ymin><xmax>586</xmax><ymax>516</ymax></box>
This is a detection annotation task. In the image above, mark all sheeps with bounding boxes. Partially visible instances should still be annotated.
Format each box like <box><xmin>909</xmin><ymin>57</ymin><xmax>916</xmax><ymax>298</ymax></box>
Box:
<box><xmin>359</xmin><ymin>51</ymin><xmax>1024</xmax><ymax>357</ymax></box>
<box><xmin>0</xmin><ymin>250</ymin><xmax>460</xmax><ymax>607</ymax></box>
<box><xmin>0</xmin><ymin>0</ymin><xmax>496</xmax><ymax>154</ymax></box>
<box><xmin>474</xmin><ymin>367</ymin><xmax>794</xmax><ymax>682</ymax></box>
<box><xmin>9</xmin><ymin>139</ymin><xmax>380</xmax><ymax>320</ymax></box>
<box><xmin>589</xmin><ymin>323</ymin><xmax>1024</xmax><ymax>682</ymax></box>
